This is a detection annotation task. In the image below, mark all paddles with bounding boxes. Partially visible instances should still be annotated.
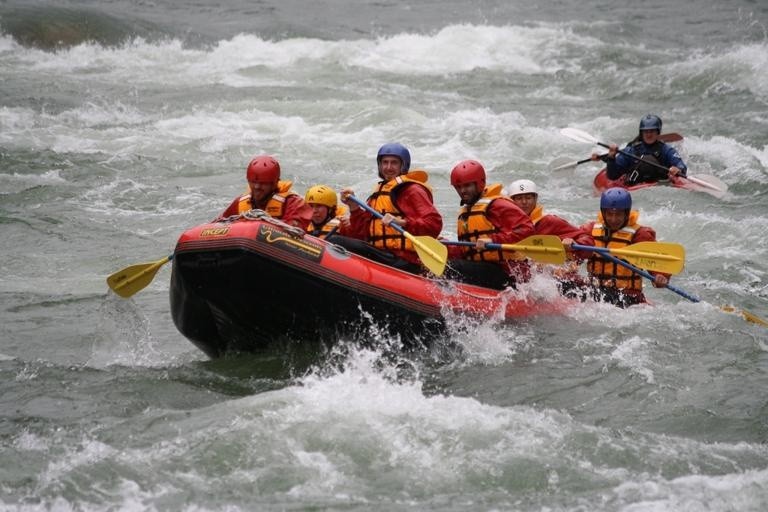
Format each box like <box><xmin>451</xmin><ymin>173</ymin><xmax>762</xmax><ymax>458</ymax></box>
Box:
<box><xmin>107</xmin><ymin>254</ymin><xmax>173</xmax><ymax>299</ymax></box>
<box><xmin>443</xmin><ymin>235</ymin><xmax>566</xmax><ymax>266</ymax></box>
<box><xmin>560</xmin><ymin>128</ymin><xmax>728</xmax><ymax>199</ymax></box>
<box><xmin>345</xmin><ymin>195</ymin><xmax>447</xmax><ymax>280</ymax></box>
<box><xmin>572</xmin><ymin>242</ymin><xmax>689</xmax><ymax>275</ymax></box>
<box><xmin>600</xmin><ymin>248</ymin><xmax>763</xmax><ymax>326</ymax></box>
<box><xmin>557</xmin><ymin>133</ymin><xmax>683</xmax><ymax>171</ymax></box>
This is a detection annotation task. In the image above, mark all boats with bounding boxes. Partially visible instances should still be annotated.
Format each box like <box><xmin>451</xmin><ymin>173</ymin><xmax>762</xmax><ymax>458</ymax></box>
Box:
<box><xmin>590</xmin><ymin>165</ymin><xmax>683</xmax><ymax>195</ymax></box>
<box><xmin>165</xmin><ymin>217</ymin><xmax>660</xmax><ymax>363</ymax></box>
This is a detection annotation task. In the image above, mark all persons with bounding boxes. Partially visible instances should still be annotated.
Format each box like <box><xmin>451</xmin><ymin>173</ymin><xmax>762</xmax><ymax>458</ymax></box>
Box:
<box><xmin>581</xmin><ymin>187</ymin><xmax>672</xmax><ymax>310</ymax></box>
<box><xmin>225</xmin><ymin>156</ymin><xmax>315</xmax><ymax>232</ymax></box>
<box><xmin>591</xmin><ymin>114</ymin><xmax>689</xmax><ymax>188</ymax></box>
<box><xmin>507</xmin><ymin>179</ymin><xmax>596</xmax><ymax>264</ymax></box>
<box><xmin>305</xmin><ymin>184</ymin><xmax>346</xmax><ymax>239</ymax></box>
<box><xmin>331</xmin><ymin>140</ymin><xmax>444</xmax><ymax>271</ymax></box>
<box><xmin>450</xmin><ymin>160</ymin><xmax>534</xmax><ymax>291</ymax></box>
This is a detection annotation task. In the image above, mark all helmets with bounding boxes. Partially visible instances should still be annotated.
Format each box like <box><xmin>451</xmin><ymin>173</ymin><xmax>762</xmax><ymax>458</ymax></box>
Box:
<box><xmin>376</xmin><ymin>144</ymin><xmax>410</xmax><ymax>179</ymax></box>
<box><xmin>509</xmin><ymin>179</ymin><xmax>537</xmax><ymax>197</ymax></box>
<box><xmin>600</xmin><ymin>188</ymin><xmax>631</xmax><ymax>210</ymax></box>
<box><xmin>451</xmin><ymin>160</ymin><xmax>485</xmax><ymax>192</ymax></box>
<box><xmin>639</xmin><ymin>115</ymin><xmax>662</xmax><ymax>130</ymax></box>
<box><xmin>305</xmin><ymin>185</ymin><xmax>337</xmax><ymax>209</ymax></box>
<box><xmin>247</xmin><ymin>156</ymin><xmax>280</xmax><ymax>190</ymax></box>
